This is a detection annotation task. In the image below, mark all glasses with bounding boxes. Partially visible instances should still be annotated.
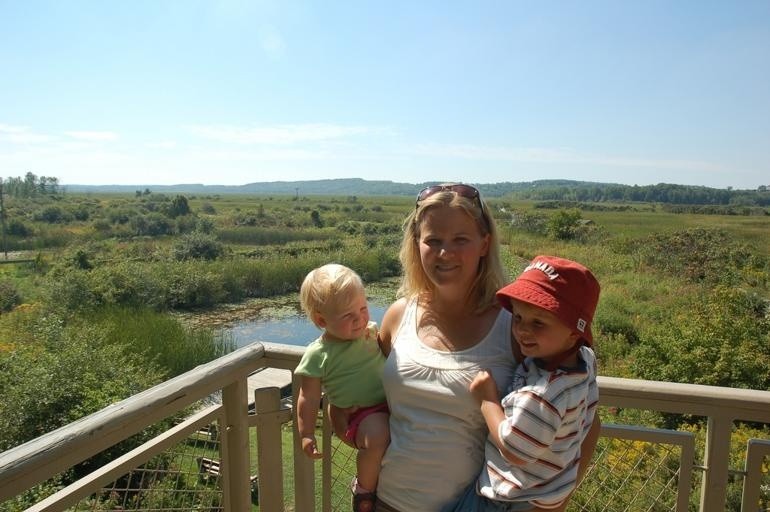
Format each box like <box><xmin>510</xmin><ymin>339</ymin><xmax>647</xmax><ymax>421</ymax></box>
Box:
<box><xmin>415</xmin><ymin>185</ymin><xmax>484</xmax><ymax>214</ymax></box>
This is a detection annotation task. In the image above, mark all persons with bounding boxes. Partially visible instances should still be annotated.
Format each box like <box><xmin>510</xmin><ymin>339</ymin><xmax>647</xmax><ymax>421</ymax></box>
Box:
<box><xmin>292</xmin><ymin>263</ymin><xmax>393</xmax><ymax>511</ymax></box>
<box><xmin>327</xmin><ymin>182</ymin><xmax>602</xmax><ymax>511</ymax></box>
<box><xmin>437</xmin><ymin>254</ymin><xmax>600</xmax><ymax>511</ymax></box>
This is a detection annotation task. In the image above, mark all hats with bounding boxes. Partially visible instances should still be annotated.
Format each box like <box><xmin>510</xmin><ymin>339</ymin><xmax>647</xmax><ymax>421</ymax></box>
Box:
<box><xmin>495</xmin><ymin>255</ymin><xmax>600</xmax><ymax>347</ymax></box>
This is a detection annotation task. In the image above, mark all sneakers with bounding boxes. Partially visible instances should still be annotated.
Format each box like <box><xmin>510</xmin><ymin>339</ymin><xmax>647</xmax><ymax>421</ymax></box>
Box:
<box><xmin>350</xmin><ymin>476</ymin><xmax>375</xmax><ymax>512</ymax></box>
